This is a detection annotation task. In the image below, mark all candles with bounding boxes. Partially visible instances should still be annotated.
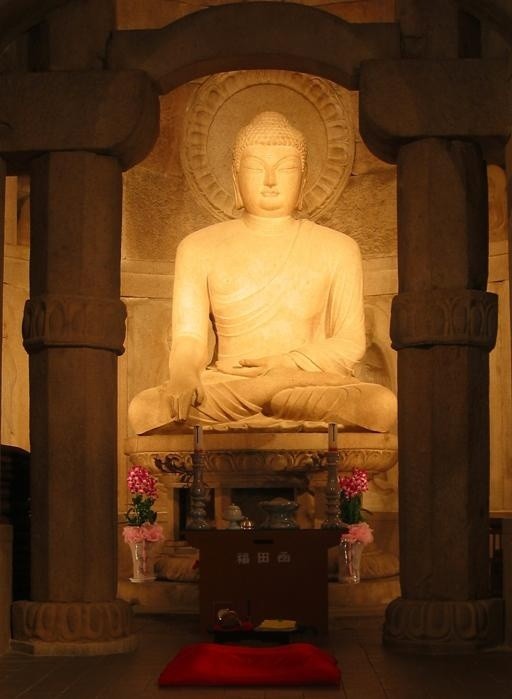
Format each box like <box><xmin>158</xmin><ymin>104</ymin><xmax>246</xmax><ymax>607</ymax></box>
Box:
<box><xmin>194</xmin><ymin>423</ymin><xmax>202</xmax><ymax>453</ymax></box>
<box><xmin>329</xmin><ymin>422</ymin><xmax>336</xmax><ymax>451</ymax></box>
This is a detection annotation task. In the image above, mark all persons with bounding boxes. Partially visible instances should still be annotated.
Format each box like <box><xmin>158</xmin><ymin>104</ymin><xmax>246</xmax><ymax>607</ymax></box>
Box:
<box><xmin>127</xmin><ymin>110</ymin><xmax>397</xmax><ymax>434</ymax></box>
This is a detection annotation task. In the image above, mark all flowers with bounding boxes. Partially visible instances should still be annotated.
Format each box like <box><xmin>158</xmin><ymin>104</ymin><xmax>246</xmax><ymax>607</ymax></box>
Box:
<box><xmin>122</xmin><ymin>464</ymin><xmax>164</xmax><ymax>573</ymax></box>
<box><xmin>334</xmin><ymin>465</ymin><xmax>374</xmax><ymax>545</ymax></box>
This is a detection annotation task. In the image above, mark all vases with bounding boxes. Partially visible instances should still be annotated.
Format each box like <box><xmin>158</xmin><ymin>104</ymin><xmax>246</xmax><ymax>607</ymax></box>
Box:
<box><xmin>339</xmin><ymin>540</ymin><xmax>364</xmax><ymax>584</ymax></box>
<box><xmin>126</xmin><ymin>536</ymin><xmax>158</xmax><ymax>583</ymax></box>
